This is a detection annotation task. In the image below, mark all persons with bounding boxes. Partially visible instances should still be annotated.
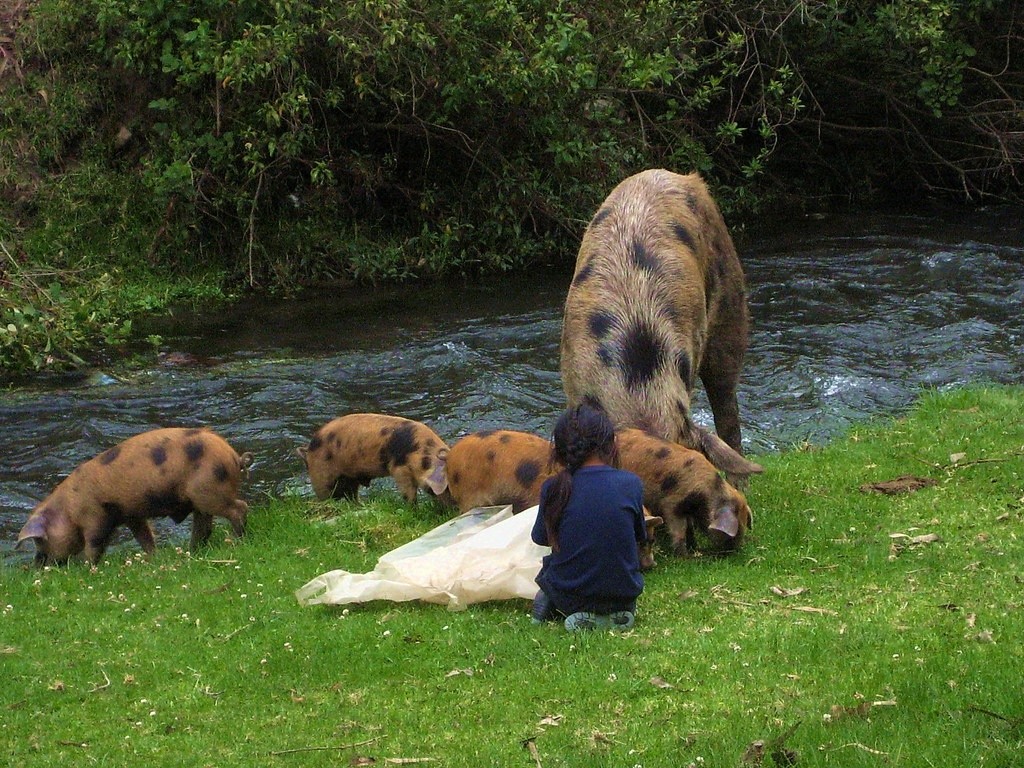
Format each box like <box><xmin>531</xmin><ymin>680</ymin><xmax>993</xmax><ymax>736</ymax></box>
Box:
<box><xmin>531</xmin><ymin>396</ymin><xmax>647</xmax><ymax>636</ymax></box>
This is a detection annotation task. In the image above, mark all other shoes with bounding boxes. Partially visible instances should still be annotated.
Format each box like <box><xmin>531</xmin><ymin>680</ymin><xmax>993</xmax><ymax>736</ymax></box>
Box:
<box><xmin>565</xmin><ymin>611</ymin><xmax>633</xmax><ymax>634</ymax></box>
<box><xmin>531</xmin><ymin>591</ymin><xmax>552</xmax><ymax>625</ymax></box>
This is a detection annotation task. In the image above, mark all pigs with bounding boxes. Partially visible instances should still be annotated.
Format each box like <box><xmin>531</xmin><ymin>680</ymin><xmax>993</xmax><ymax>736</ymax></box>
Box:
<box><xmin>613</xmin><ymin>426</ymin><xmax>754</xmax><ymax>557</ymax></box>
<box><xmin>16</xmin><ymin>425</ymin><xmax>247</xmax><ymax>570</ymax></box>
<box><xmin>436</xmin><ymin>429</ymin><xmax>664</xmax><ymax>568</ymax></box>
<box><xmin>297</xmin><ymin>414</ymin><xmax>452</xmax><ymax>505</ymax></box>
<box><xmin>560</xmin><ymin>169</ymin><xmax>765</xmax><ymax>492</ymax></box>
